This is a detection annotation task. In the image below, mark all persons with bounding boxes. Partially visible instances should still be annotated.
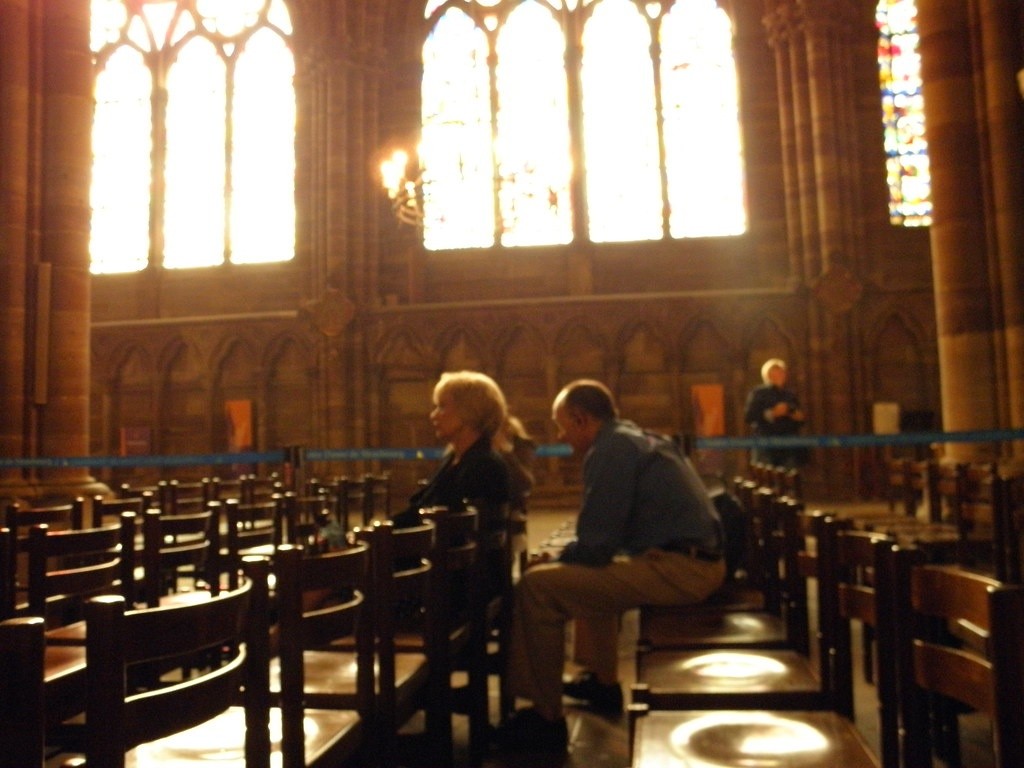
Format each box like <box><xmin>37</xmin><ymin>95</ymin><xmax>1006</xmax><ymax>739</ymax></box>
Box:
<box><xmin>262</xmin><ymin>366</ymin><xmax>526</xmax><ymax>660</ymax></box>
<box><xmin>494</xmin><ymin>377</ymin><xmax>725</xmax><ymax>753</ymax></box>
<box><xmin>744</xmin><ymin>359</ymin><xmax>808</xmax><ymax>490</ymax></box>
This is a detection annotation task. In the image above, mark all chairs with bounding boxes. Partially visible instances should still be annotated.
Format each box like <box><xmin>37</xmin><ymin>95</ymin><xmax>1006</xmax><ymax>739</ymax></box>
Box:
<box><xmin>0</xmin><ymin>450</ymin><xmax>1024</xmax><ymax>768</ymax></box>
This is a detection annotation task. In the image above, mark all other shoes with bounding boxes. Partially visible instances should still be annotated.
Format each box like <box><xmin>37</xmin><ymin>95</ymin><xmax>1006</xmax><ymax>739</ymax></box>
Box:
<box><xmin>562</xmin><ymin>669</ymin><xmax>622</xmax><ymax>714</ymax></box>
<box><xmin>495</xmin><ymin>707</ymin><xmax>568</xmax><ymax>750</ymax></box>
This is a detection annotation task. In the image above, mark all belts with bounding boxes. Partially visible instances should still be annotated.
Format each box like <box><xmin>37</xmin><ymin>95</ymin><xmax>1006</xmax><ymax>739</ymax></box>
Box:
<box><xmin>660</xmin><ymin>544</ymin><xmax>722</xmax><ymax>561</ymax></box>
